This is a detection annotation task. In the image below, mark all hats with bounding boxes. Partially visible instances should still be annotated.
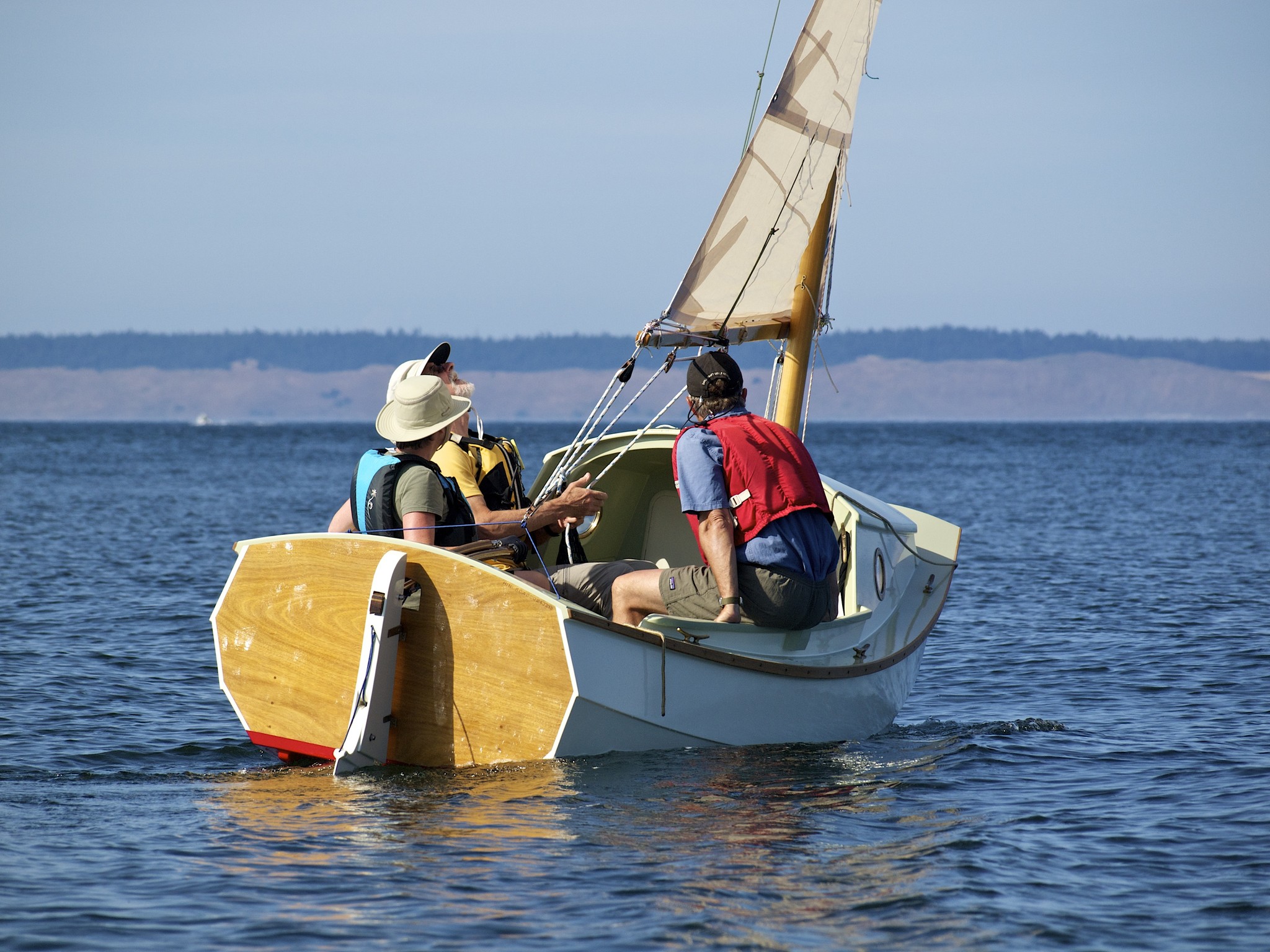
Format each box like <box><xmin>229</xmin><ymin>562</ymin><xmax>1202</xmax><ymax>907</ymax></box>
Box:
<box><xmin>686</xmin><ymin>351</ymin><xmax>743</xmax><ymax>398</ymax></box>
<box><xmin>386</xmin><ymin>342</ymin><xmax>450</xmax><ymax>403</ymax></box>
<box><xmin>376</xmin><ymin>374</ymin><xmax>472</xmax><ymax>442</ymax></box>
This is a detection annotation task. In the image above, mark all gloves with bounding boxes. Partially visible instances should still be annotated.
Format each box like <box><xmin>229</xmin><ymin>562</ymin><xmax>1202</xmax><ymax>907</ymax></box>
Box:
<box><xmin>497</xmin><ymin>535</ymin><xmax>528</xmax><ymax>565</ymax></box>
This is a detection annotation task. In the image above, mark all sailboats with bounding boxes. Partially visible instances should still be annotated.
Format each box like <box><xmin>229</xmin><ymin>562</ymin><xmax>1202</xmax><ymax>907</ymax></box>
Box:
<box><xmin>208</xmin><ymin>1</ymin><xmax>964</xmax><ymax>769</ymax></box>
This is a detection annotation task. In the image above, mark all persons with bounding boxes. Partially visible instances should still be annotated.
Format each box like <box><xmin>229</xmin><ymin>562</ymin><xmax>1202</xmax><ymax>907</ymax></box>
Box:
<box><xmin>611</xmin><ymin>350</ymin><xmax>839</xmax><ymax>627</ymax></box>
<box><xmin>386</xmin><ymin>342</ymin><xmax>660</xmax><ymax>620</ymax></box>
<box><xmin>328</xmin><ymin>374</ymin><xmax>552</xmax><ymax>591</ymax></box>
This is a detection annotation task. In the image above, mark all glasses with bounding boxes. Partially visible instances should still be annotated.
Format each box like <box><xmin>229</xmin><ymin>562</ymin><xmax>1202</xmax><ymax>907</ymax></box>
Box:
<box><xmin>429</xmin><ymin>362</ymin><xmax>444</xmax><ymax>375</ymax></box>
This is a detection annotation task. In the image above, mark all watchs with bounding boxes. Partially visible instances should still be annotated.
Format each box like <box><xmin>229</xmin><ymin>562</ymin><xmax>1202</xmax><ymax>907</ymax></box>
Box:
<box><xmin>719</xmin><ymin>597</ymin><xmax>743</xmax><ymax>610</ymax></box>
